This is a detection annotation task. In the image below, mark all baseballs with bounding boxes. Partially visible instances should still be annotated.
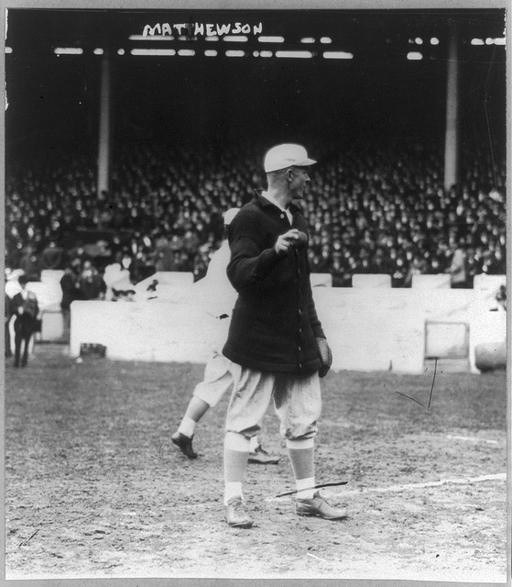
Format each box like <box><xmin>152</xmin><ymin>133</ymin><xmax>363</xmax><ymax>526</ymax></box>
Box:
<box><xmin>292</xmin><ymin>231</ymin><xmax>308</xmax><ymax>249</ymax></box>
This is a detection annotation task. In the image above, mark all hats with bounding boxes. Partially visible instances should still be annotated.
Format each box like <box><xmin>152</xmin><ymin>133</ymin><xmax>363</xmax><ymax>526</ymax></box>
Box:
<box><xmin>262</xmin><ymin>141</ymin><xmax>318</xmax><ymax>174</ymax></box>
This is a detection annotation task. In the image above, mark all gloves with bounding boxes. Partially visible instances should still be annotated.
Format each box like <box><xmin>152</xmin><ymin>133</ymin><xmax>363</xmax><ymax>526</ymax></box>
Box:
<box><xmin>317</xmin><ymin>338</ymin><xmax>333</xmax><ymax>378</ymax></box>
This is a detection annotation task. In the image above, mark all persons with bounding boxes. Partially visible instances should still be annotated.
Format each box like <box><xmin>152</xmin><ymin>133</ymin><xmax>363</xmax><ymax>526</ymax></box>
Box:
<box><xmin>59</xmin><ymin>263</ymin><xmax>82</xmax><ymax>340</ymax></box>
<box><xmin>168</xmin><ymin>204</ymin><xmax>285</xmax><ymax>467</ymax></box>
<box><xmin>1</xmin><ymin>132</ymin><xmax>506</xmax><ymax>300</ymax></box>
<box><xmin>223</xmin><ymin>141</ymin><xmax>350</xmax><ymax>529</ymax></box>
<box><xmin>11</xmin><ymin>275</ymin><xmax>39</xmax><ymax>367</ymax></box>
<box><xmin>5</xmin><ymin>291</ymin><xmax>13</xmax><ymax>358</ymax></box>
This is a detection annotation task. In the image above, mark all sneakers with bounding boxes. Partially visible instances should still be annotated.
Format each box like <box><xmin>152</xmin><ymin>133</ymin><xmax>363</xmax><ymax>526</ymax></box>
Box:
<box><xmin>221</xmin><ymin>495</ymin><xmax>255</xmax><ymax>528</ymax></box>
<box><xmin>294</xmin><ymin>491</ymin><xmax>348</xmax><ymax>520</ymax></box>
<box><xmin>171</xmin><ymin>430</ymin><xmax>198</xmax><ymax>460</ymax></box>
<box><xmin>247</xmin><ymin>444</ymin><xmax>282</xmax><ymax>464</ymax></box>
